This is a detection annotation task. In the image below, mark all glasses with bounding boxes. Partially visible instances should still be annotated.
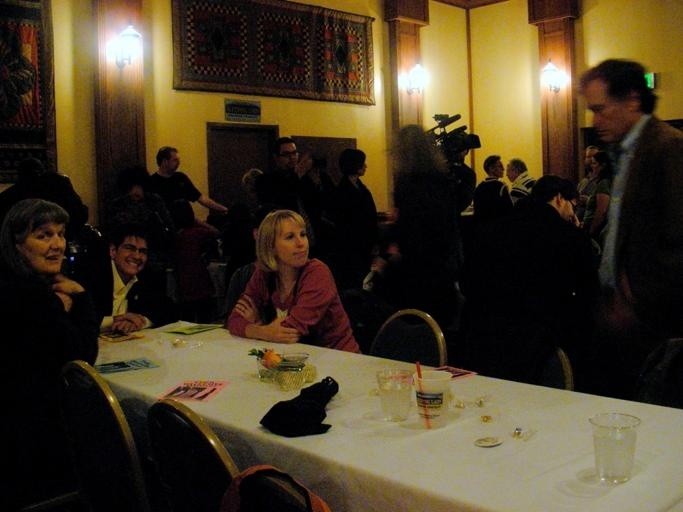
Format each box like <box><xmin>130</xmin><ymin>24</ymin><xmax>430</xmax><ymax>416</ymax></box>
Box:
<box><xmin>277</xmin><ymin>151</ymin><xmax>298</xmax><ymax>157</ymax></box>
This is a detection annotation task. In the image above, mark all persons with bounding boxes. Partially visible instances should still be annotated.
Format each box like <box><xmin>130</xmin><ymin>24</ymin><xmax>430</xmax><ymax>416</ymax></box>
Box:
<box><xmin>0</xmin><ymin>146</ymin><xmax>238</xmax><ymax>512</ymax></box>
<box><xmin>228</xmin><ymin>55</ymin><xmax>683</xmax><ymax>407</ymax></box>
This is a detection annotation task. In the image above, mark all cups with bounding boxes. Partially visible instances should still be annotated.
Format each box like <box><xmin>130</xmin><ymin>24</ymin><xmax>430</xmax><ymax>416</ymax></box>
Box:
<box><xmin>588</xmin><ymin>411</ymin><xmax>640</xmax><ymax>481</ymax></box>
<box><xmin>412</xmin><ymin>371</ymin><xmax>454</xmax><ymax>428</ymax></box>
<box><xmin>375</xmin><ymin>366</ymin><xmax>413</xmax><ymax>420</ymax></box>
<box><xmin>258</xmin><ymin>350</ymin><xmax>316</xmax><ymax>391</ymax></box>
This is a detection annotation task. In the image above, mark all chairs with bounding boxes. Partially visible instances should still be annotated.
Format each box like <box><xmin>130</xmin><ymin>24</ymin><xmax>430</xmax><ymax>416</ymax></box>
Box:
<box><xmin>537</xmin><ymin>345</ymin><xmax>574</xmax><ymax>391</ymax></box>
<box><xmin>64</xmin><ymin>354</ymin><xmax>137</xmax><ymax>495</ymax></box>
<box><xmin>236</xmin><ymin>463</ymin><xmax>325</xmax><ymax>512</ymax></box>
<box><xmin>369</xmin><ymin>308</ymin><xmax>448</xmax><ymax>368</ymax></box>
<box><xmin>136</xmin><ymin>397</ymin><xmax>240</xmax><ymax>511</ymax></box>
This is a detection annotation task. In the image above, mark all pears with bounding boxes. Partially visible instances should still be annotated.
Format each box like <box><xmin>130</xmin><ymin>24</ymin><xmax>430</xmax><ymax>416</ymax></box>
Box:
<box><xmin>261</xmin><ymin>349</ymin><xmax>282</xmax><ymax>369</ymax></box>
